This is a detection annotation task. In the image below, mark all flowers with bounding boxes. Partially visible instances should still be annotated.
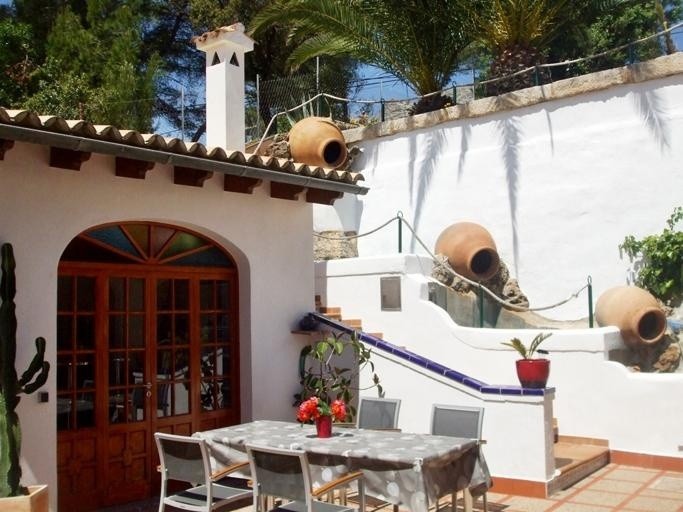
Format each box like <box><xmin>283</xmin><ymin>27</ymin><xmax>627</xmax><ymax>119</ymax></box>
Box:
<box><xmin>297</xmin><ymin>398</ymin><xmax>347</xmax><ymax>427</ymax></box>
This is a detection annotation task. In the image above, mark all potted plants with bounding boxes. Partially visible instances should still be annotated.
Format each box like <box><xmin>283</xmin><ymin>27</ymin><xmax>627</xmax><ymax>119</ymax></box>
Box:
<box><xmin>1</xmin><ymin>242</ymin><xmax>51</xmax><ymax>512</ymax></box>
<box><xmin>499</xmin><ymin>332</ymin><xmax>555</xmax><ymax>389</ymax></box>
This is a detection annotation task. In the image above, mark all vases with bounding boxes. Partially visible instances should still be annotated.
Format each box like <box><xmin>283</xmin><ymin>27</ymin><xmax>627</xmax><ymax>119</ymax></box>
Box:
<box><xmin>314</xmin><ymin>416</ymin><xmax>333</xmax><ymax>438</ymax></box>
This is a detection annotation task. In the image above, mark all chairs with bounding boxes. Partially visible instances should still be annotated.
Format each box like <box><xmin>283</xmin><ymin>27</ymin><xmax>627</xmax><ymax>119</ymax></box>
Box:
<box><xmin>355</xmin><ymin>397</ymin><xmax>402</xmax><ymax>432</ymax></box>
<box><xmin>244</xmin><ymin>444</ymin><xmax>364</xmax><ymax>512</ymax></box>
<box><xmin>429</xmin><ymin>404</ymin><xmax>488</xmax><ymax>512</ymax></box>
<box><xmin>153</xmin><ymin>433</ymin><xmax>251</xmax><ymax>512</ymax></box>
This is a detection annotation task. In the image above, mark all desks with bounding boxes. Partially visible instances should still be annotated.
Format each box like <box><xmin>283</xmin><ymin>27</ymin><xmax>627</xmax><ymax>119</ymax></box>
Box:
<box><xmin>192</xmin><ymin>419</ymin><xmax>494</xmax><ymax>512</ymax></box>
